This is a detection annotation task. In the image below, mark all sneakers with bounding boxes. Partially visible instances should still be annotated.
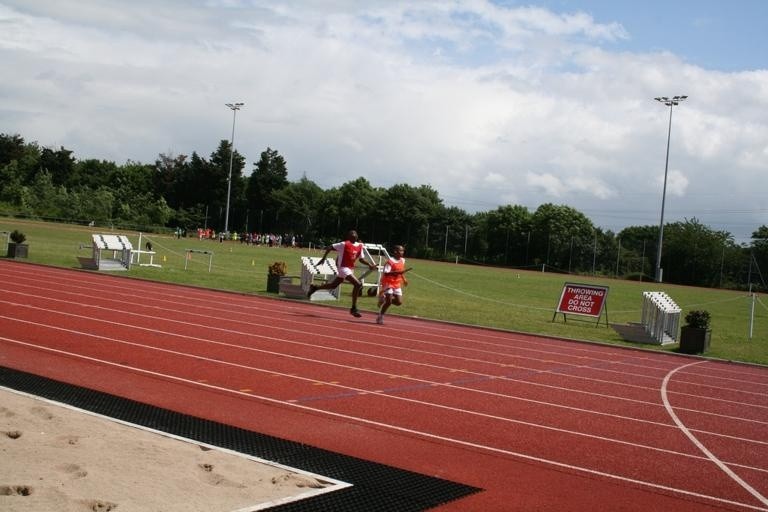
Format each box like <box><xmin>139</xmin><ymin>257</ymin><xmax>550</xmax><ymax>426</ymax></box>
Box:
<box><xmin>350</xmin><ymin>308</ymin><xmax>362</xmax><ymax>317</ymax></box>
<box><xmin>377</xmin><ymin>296</ymin><xmax>384</xmax><ymax>308</ymax></box>
<box><xmin>307</xmin><ymin>284</ymin><xmax>316</xmax><ymax>297</ymax></box>
<box><xmin>376</xmin><ymin>315</ymin><xmax>384</xmax><ymax>324</ymax></box>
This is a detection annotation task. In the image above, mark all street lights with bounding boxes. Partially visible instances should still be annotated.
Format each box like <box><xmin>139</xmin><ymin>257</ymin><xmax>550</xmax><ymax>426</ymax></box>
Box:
<box><xmin>651</xmin><ymin>93</ymin><xmax>689</xmax><ymax>283</ymax></box>
<box><xmin>223</xmin><ymin>102</ymin><xmax>246</xmax><ymax>231</ymax></box>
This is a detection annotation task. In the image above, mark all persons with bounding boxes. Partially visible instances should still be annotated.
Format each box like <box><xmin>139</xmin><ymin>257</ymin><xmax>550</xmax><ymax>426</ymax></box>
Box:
<box><xmin>173</xmin><ymin>223</ymin><xmax>306</xmax><ymax>249</ymax></box>
<box><xmin>306</xmin><ymin>228</ymin><xmax>380</xmax><ymax>318</ymax></box>
<box><xmin>145</xmin><ymin>239</ymin><xmax>153</xmax><ymax>252</ymax></box>
<box><xmin>375</xmin><ymin>244</ymin><xmax>412</xmax><ymax>325</ymax></box>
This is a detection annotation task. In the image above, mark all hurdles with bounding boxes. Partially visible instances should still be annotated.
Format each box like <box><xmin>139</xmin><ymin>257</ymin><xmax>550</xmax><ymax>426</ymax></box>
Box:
<box><xmin>185</xmin><ymin>249</ymin><xmax>213</xmax><ymax>272</ymax></box>
<box><xmin>300</xmin><ymin>256</ymin><xmax>341</xmax><ymax>302</ymax></box>
<box><xmin>351</xmin><ymin>243</ymin><xmax>390</xmax><ymax>287</ymax></box>
<box><xmin>642</xmin><ymin>291</ymin><xmax>682</xmax><ymax>346</ymax></box>
<box><xmin>92</xmin><ymin>234</ymin><xmax>133</xmax><ymax>271</ymax></box>
